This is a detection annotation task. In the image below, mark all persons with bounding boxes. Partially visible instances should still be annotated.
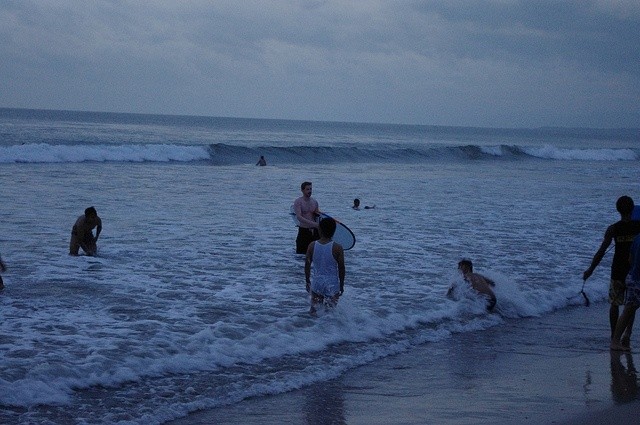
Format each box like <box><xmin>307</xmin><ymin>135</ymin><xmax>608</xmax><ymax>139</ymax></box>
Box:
<box><xmin>294</xmin><ymin>181</ymin><xmax>321</xmax><ymax>254</ymax></box>
<box><xmin>255</xmin><ymin>156</ymin><xmax>266</xmax><ymax>166</ymax></box>
<box><xmin>70</xmin><ymin>206</ymin><xmax>102</xmax><ymax>256</ymax></box>
<box><xmin>305</xmin><ymin>217</ymin><xmax>346</xmax><ymax>313</ymax></box>
<box><xmin>352</xmin><ymin>199</ymin><xmax>361</xmax><ymax>209</ymax></box>
<box><xmin>610</xmin><ymin>234</ymin><xmax>640</xmax><ymax>351</ymax></box>
<box><xmin>445</xmin><ymin>260</ymin><xmax>497</xmax><ymax>311</ymax></box>
<box><xmin>583</xmin><ymin>195</ymin><xmax>640</xmax><ymax>338</ymax></box>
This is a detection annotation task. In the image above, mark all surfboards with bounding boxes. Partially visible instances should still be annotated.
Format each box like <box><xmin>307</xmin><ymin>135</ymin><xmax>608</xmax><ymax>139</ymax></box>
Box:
<box><xmin>289</xmin><ymin>205</ymin><xmax>356</xmax><ymax>250</ymax></box>
